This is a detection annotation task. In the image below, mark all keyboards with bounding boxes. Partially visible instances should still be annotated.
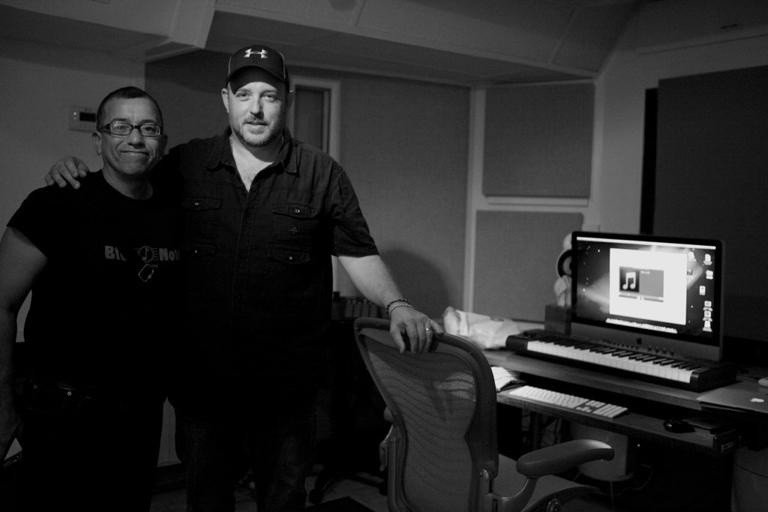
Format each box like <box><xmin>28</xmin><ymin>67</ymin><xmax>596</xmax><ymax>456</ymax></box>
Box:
<box><xmin>509</xmin><ymin>384</ymin><xmax>628</xmax><ymax>419</ymax></box>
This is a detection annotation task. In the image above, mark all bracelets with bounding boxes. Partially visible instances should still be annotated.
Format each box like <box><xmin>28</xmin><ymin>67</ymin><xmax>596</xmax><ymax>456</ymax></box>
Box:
<box><xmin>388</xmin><ymin>304</ymin><xmax>412</xmax><ymax>319</ymax></box>
<box><xmin>385</xmin><ymin>298</ymin><xmax>407</xmax><ymax>312</ymax></box>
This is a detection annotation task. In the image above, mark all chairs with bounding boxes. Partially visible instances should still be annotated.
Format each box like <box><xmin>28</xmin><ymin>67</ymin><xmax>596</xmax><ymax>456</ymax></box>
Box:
<box><xmin>354</xmin><ymin>316</ymin><xmax>614</xmax><ymax>512</ymax></box>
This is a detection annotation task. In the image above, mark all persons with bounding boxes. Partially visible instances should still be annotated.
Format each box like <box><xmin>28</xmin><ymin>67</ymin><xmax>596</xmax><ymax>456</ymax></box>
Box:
<box><xmin>0</xmin><ymin>86</ymin><xmax>190</xmax><ymax>512</ymax></box>
<box><xmin>44</xmin><ymin>45</ymin><xmax>445</xmax><ymax>512</ymax></box>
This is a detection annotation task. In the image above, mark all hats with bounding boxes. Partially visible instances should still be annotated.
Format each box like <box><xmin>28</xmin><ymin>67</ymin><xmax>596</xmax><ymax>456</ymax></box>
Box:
<box><xmin>224</xmin><ymin>44</ymin><xmax>289</xmax><ymax>87</ymax></box>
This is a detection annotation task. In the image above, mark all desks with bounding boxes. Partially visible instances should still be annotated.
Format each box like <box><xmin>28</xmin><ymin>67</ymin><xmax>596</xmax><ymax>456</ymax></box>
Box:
<box><xmin>481</xmin><ymin>347</ymin><xmax>768</xmax><ymax>459</ymax></box>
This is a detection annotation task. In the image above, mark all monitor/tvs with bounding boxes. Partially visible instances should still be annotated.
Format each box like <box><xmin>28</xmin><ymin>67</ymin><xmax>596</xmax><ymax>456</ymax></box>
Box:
<box><xmin>569</xmin><ymin>230</ymin><xmax>725</xmax><ymax>365</ymax></box>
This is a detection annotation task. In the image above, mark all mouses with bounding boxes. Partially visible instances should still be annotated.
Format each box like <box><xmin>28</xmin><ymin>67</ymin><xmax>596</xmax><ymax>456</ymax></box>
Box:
<box><xmin>663</xmin><ymin>419</ymin><xmax>696</xmax><ymax>433</ymax></box>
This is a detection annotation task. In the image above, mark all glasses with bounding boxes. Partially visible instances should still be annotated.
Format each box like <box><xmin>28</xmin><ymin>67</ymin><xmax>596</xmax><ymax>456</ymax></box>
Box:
<box><xmin>97</xmin><ymin>120</ymin><xmax>162</xmax><ymax>137</ymax></box>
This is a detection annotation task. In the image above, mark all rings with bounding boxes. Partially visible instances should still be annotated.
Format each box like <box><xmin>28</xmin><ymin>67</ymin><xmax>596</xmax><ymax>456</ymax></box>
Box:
<box><xmin>425</xmin><ymin>327</ymin><xmax>431</xmax><ymax>333</ymax></box>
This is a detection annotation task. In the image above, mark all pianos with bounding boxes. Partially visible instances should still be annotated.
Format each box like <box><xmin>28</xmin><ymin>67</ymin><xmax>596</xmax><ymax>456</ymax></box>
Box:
<box><xmin>505</xmin><ymin>327</ymin><xmax>712</xmax><ymax>391</ymax></box>
